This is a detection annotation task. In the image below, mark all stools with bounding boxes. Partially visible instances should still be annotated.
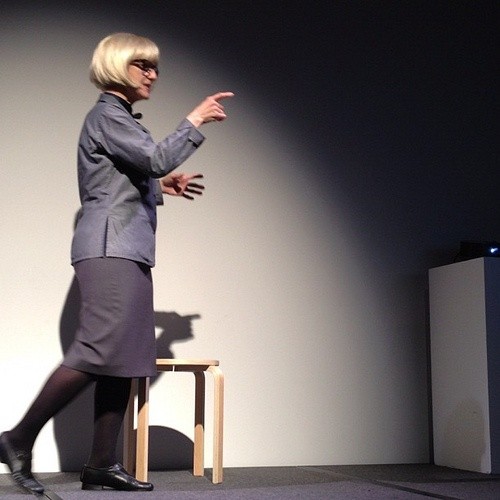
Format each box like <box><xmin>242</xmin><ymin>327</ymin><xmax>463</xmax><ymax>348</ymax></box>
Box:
<box><xmin>122</xmin><ymin>358</ymin><xmax>224</xmax><ymax>485</ymax></box>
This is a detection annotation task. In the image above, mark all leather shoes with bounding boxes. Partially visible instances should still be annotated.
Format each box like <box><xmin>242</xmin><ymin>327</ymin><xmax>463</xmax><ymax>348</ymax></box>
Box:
<box><xmin>0</xmin><ymin>430</ymin><xmax>44</xmax><ymax>494</ymax></box>
<box><xmin>80</xmin><ymin>462</ymin><xmax>154</xmax><ymax>491</ymax></box>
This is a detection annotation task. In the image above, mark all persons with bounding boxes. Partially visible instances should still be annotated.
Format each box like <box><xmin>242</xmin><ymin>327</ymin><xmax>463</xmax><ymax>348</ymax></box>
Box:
<box><xmin>0</xmin><ymin>32</ymin><xmax>235</xmax><ymax>494</ymax></box>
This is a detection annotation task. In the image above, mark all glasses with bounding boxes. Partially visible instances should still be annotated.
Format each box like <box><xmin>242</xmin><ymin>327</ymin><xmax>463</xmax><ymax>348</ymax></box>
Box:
<box><xmin>130</xmin><ymin>60</ymin><xmax>160</xmax><ymax>75</ymax></box>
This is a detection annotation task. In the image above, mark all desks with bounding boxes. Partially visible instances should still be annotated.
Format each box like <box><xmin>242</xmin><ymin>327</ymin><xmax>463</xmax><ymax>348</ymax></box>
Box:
<box><xmin>427</xmin><ymin>257</ymin><xmax>500</xmax><ymax>476</ymax></box>
